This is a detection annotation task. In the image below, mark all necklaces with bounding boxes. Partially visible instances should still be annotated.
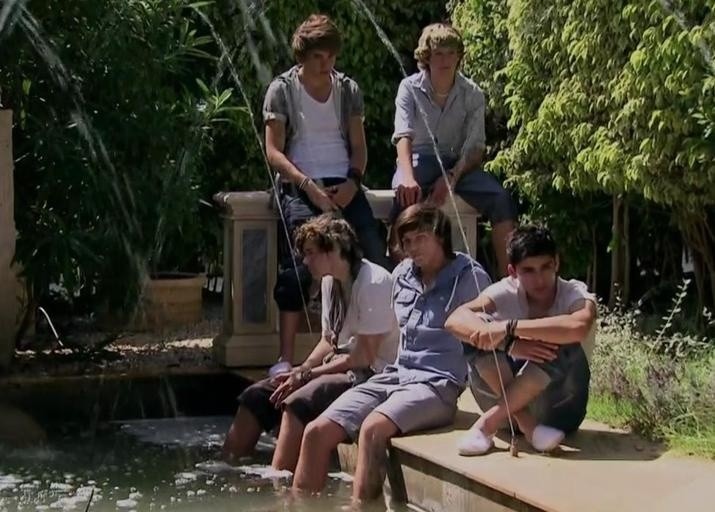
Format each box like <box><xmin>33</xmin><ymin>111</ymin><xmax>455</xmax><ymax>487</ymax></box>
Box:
<box><xmin>432</xmin><ymin>89</ymin><xmax>451</xmax><ymax>101</ymax></box>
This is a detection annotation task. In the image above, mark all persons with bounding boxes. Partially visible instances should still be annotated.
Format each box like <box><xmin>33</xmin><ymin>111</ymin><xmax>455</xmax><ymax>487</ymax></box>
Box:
<box><xmin>387</xmin><ymin>22</ymin><xmax>521</xmax><ymax>282</ymax></box>
<box><xmin>215</xmin><ymin>211</ymin><xmax>400</xmax><ymax>480</ymax></box>
<box><xmin>257</xmin><ymin>203</ymin><xmax>494</xmax><ymax>511</ymax></box>
<box><xmin>441</xmin><ymin>224</ymin><xmax>599</xmax><ymax>456</ymax></box>
<box><xmin>261</xmin><ymin>10</ymin><xmax>392</xmax><ymax>380</ymax></box>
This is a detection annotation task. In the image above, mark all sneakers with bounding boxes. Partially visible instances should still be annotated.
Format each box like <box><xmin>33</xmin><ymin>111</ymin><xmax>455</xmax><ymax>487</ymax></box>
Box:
<box><xmin>525</xmin><ymin>422</ymin><xmax>565</xmax><ymax>452</ymax></box>
<box><xmin>268</xmin><ymin>360</ymin><xmax>294</xmax><ymax>379</ymax></box>
<box><xmin>455</xmin><ymin>427</ymin><xmax>498</xmax><ymax>456</ymax></box>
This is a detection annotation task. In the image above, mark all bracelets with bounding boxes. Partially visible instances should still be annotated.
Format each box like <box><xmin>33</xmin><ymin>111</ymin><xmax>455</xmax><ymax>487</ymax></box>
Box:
<box><xmin>346</xmin><ymin>167</ymin><xmax>363</xmax><ymax>181</ymax></box>
<box><xmin>298</xmin><ymin>176</ymin><xmax>310</xmax><ymax>192</ymax></box>
<box><xmin>299</xmin><ymin>369</ymin><xmax>314</xmax><ymax>385</ymax></box>
<box><xmin>505</xmin><ymin>317</ymin><xmax>518</xmax><ymax>337</ymax></box>
<box><xmin>447</xmin><ymin>167</ymin><xmax>460</xmax><ymax>181</ymax></box>
